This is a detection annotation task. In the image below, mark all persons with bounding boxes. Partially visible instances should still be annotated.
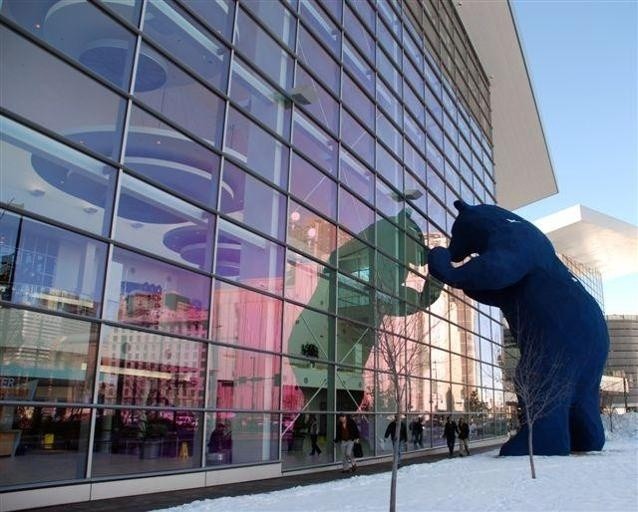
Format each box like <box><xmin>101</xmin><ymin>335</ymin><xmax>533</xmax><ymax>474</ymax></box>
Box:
<box><xmin>440</xmin><ymin>414</ymin><xmax>459</xmax><ymax>458</ymax></box>
<box><xmin>207</xmin><ymin>423</ymin><xmax>228</xmax><ymax>465</ymax></box>
<box><xmin>334</xmin><ymin>414</ymin><xmax>360</xmax><ymax>473</ymax></box>
<box><xmin>282</xmin><ymin>417</ymin><xmax>293</xmax><ymax>452</ymax></box>
<box><xmin>409</xmin><ymin>417</ymin><xmax>424</xmax><ymax>449</ymax></box>
<box><xmin>308</xmin><ymin>415</ymin><xmax>322</xmax><ymax>457</ymax></box>
<box><xmin>383</xmin><ymin>413</ymin><xmax>408</xmax><ymax>469</ymax></box>
<box><xmin>456</xmin><ymin>417</ymin><xmax>471</xmax><ymax>457</ymax></box>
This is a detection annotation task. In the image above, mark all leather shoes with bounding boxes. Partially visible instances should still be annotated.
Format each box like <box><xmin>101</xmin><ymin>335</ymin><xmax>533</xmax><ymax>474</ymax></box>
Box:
<box><xmin>342</xmin><ymin>464</ymin><xmax>356</xmax><ymax>472</ymax></box>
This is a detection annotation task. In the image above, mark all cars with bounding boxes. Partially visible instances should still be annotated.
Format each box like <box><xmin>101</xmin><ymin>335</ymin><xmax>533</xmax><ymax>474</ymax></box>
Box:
<box><xmin>471</xmin><ymin>421</ymin><xmax>507</xmax><ymax>438</ymax></box>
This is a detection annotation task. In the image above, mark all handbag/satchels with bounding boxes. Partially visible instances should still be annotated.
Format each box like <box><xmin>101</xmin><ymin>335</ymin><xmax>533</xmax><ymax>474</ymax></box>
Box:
<box><xmin>353</xmin><ymin>443</ymin><xmax>363</xmax><ymax>457</ymax></box>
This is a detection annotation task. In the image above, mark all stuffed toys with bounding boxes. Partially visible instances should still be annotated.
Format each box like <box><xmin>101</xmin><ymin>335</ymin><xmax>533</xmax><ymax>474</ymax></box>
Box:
<box><xmin>427</xmin><ymin>198</ymin><xmax>610</xmax><ymax>457</ymax></box>
<box><xmin>287</xmin><ymin>208</ymin><xmax>444</xmax><ymax>436</ymax></box>
